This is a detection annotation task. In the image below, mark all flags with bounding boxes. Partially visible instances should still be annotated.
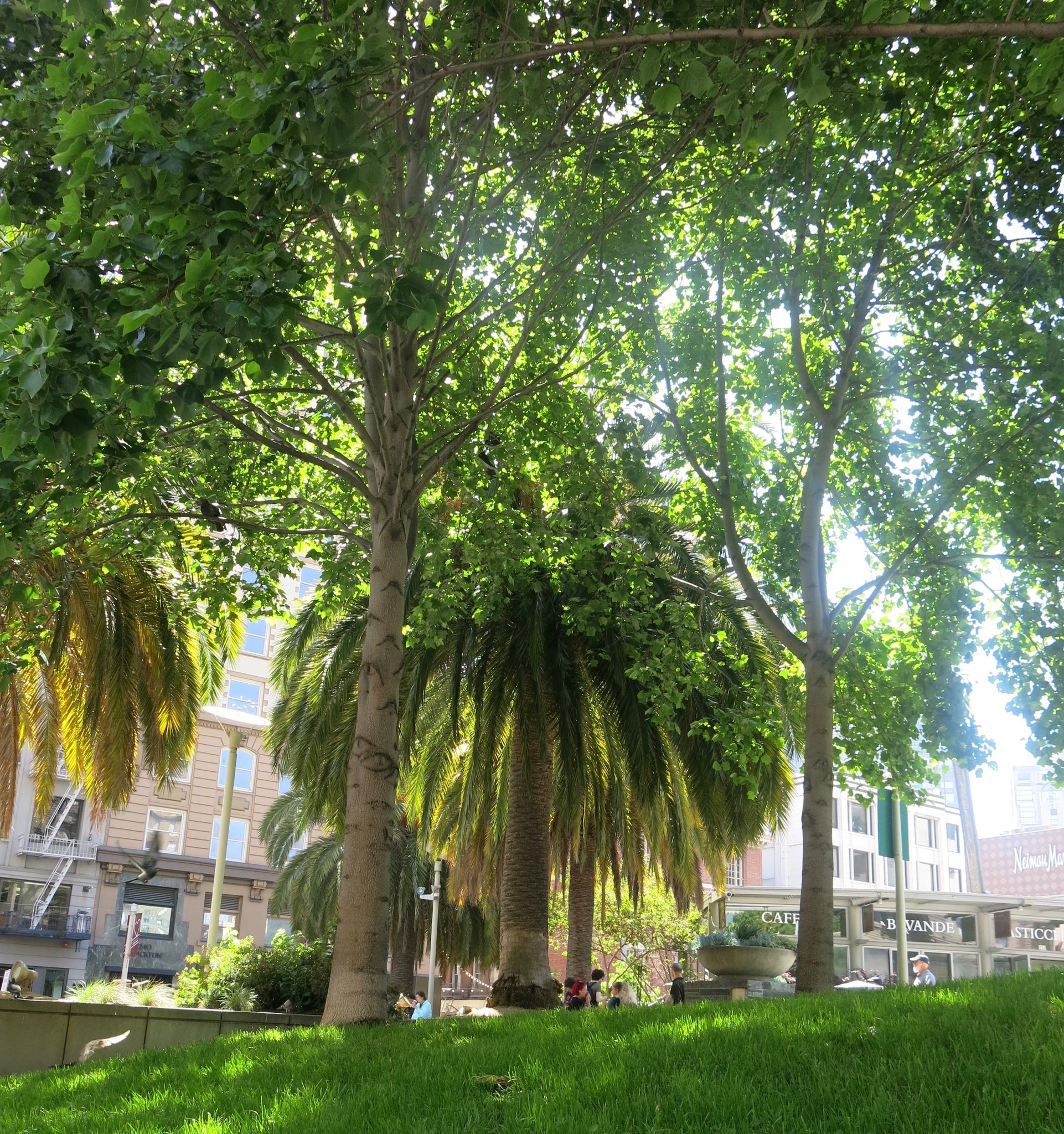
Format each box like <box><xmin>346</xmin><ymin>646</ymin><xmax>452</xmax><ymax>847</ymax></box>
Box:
<box><xmin>123</xmin><ymin>913</ymin><xmax>143</xmax><ymax>958</ymax></box>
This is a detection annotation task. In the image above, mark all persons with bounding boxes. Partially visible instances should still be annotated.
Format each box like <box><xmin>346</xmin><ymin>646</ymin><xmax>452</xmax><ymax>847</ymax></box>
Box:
<box><xmin>909</xmin><ymin>954</ymin><xmax>935</xmax><ymax>988</ymax></box>
<box><xmin>564</xmin><ymin>976</ymin><xmax>576</xmax><ymax>1011</ymax></box>
<box><xmin>867</xmin><ymin>976</ymin><xmax>882</xmax><ymax>986</ymax></box>
<box><xmin>568</xmin><ymin>979</ymin><xmax>591</xmax><ymax>1012</ymax></box>
<box><xmin>669</xmin><ymin>963</ymin><xmax>685</xmax><ymax>1006</ymax></box>
<box><xmin>411</xmin><ymin>991</ymin><xmax>432</xmax><ymax>1020</ymax></box>
<box><xmin>604</xmin><ymin>982</ymin><xmax>637</xmax><ymax>1009</ymax></box>
<box><xmin>588</xmin><ymin>969</ymin><xmax>608</xmax><ymax>1008</ymax></box>
<box><xmin>403</xmin><ymin>991</ymin><xmax>417</xmax><ymax>1022</ymax></box>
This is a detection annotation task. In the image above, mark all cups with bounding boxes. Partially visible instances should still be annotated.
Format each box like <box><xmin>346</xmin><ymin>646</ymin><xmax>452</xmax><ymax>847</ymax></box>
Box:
<box><xmin>411</xmin><ymin>1000</ymin><xmax>417</xmax><ymax>1008</ymax></box>
